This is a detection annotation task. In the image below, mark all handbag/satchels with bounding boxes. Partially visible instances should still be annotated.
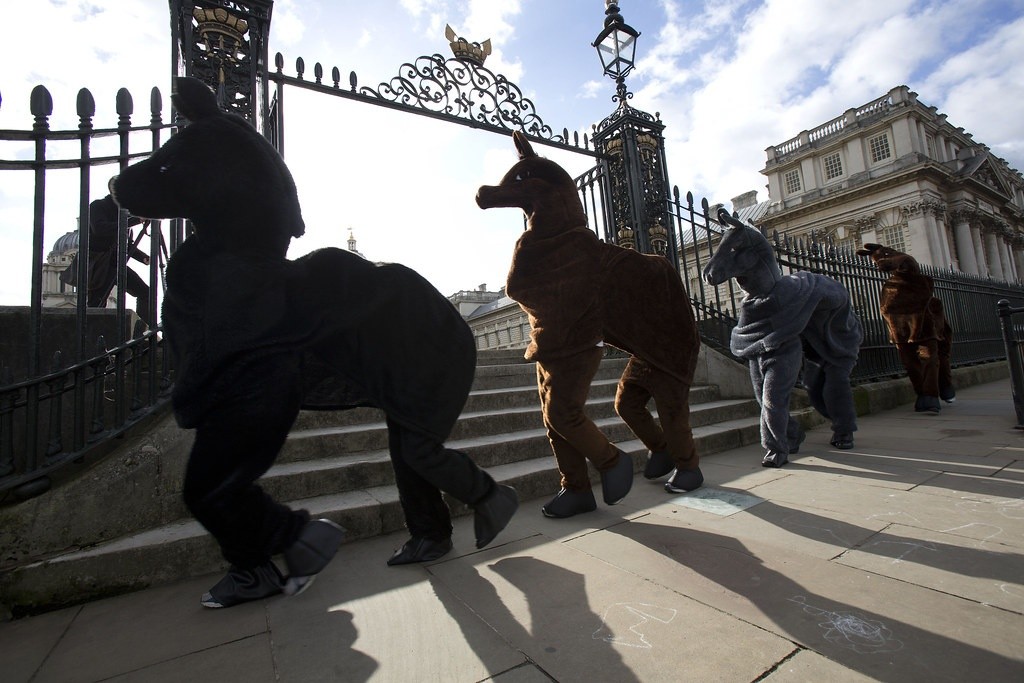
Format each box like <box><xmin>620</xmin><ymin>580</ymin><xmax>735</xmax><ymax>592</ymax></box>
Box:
<box><xmin>60</xmin><ymin>249</ymin><xmax>112</xmax><ymax>290</ymax></box>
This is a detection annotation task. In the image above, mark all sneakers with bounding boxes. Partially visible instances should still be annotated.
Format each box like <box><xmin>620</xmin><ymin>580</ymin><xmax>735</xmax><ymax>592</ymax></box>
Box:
<box><xmin>201</xmin><ymin>560</ymin><xmax>281</xmax><ymax>607</ymax></box>
<box><xmin>284</xmin><ymin>517</ymin><xmax>335</xmax><ymax>598</ymax></box>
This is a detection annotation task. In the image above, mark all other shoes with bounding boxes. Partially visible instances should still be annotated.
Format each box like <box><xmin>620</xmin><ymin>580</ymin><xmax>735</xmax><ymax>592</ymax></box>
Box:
<box><xmin>388</xmin><ymin>540</ymin><xmax>453</xmax><ymax>563</ymax></box>
<box><xmin>664</xmin><ymin>466</ymin><xmax>704</xmax><ymax>494</ymax></box>
<box><xmin>918</xmin><ymin>401</ymin><xmax>939</xmax><ymax>413</ymax></box>
<box><xmin>788</xmin><ymin>445</ymin><xmax>799</xmax><ymax>453</ymax></box>
<box><xmin>473</xmin><ymin>484</ymin><xmax>512</xmax><ymax>548</ymax></box>
<box><xmin>942</xmin><ymin>395</ymin><xmax>956</xmax><ymax>402</ymax></box>
<box><xmin>832</xmin><ymin>438</ymin><xmax>855</xmax><ymax>449</ymax></box>
<box><xmin>602</xmin><ymin>452</ymin><xmax>633</xmax><ymax>504</ymax></box>
<box><xmin>539</xmin><ymin>483</ymin><xmax>598</xmax><ymax>519</ymax></box>
<box><xmin>644</xmin><ymin>448</ymin><xmax>677</xmax><ymax>480</ymax></box>
<box><xmin>762</xmin><ymin>450</ymin><xmax>787</xmax><ymax>467</ymax></box>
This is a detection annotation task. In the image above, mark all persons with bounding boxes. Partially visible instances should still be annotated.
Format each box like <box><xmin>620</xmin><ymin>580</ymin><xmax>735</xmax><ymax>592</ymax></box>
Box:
<box><xmin>59</xmin><ymin>175</ymin><xmax>153</xmax><ymax>358</ymax></box>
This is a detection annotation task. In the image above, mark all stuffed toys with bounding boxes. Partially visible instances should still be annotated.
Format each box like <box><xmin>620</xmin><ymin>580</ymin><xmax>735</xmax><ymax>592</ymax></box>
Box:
<box><xmin>475</xmin><ymin>131</ymin><xmax>705</xmax><ymax>520</ymax></box>
<box><xmin>703</xmin><ymin>208</ymin><xmax>866</xmax><ymax>469</ymax></box>
<box><xmin>108</xmin><ymin>75</ymin><xmax>521</xmax><ymax>609</ymax></box>
<box><xmin>853</xmin><ymin>242</ymin><xmax>957</xmax><ymax>414</ymax></box>
